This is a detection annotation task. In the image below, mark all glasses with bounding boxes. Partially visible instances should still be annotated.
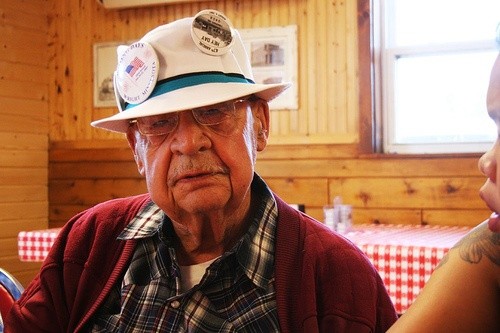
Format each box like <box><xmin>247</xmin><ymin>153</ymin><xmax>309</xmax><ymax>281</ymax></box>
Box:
<box><xmin>128</xmin><ymin>98</ymin><xmax>260</xmax><ymax>136</ymax></box>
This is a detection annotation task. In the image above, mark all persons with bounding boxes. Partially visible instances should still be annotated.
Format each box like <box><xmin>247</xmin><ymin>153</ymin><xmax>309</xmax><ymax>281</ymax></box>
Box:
<box><xmin>370</xmin><ymin>41</ymin><xmax>499</xmax><ymax>333</ymax></box>
<box><xmin>0</xmin><ymin>9</ymin><xmax>398</xmax><ymax>332</ymax></box>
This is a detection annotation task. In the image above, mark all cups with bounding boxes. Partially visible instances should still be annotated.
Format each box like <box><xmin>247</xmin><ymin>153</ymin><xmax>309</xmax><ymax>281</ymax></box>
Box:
<box><xmin>324</xmin><ymin>207</ymin><xmax>338</xmax><ymax>232</ymax></box>
<box><xmin>339</xmin><ymin>206</ymin><xmax>354</xmax><ymax>233</ymax></box>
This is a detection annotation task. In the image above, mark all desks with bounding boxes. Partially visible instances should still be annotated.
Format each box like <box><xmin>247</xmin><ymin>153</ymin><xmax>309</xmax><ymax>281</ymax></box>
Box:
<box><xmin>18</xmin><ymin>221</ymin><xmax>476</xmax><ymax>319</ymax></box>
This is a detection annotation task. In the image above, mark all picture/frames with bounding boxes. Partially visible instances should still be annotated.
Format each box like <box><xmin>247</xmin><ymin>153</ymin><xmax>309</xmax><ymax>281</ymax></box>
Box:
<box><xmin>92</xmin><ymin>38</ymin><xmax>135</xmax><ymax>109</ymax></box>
<box><xmin>238</xmin><ymin>24</ymin><xmax>299</xmax><ymax>113</ymax></box>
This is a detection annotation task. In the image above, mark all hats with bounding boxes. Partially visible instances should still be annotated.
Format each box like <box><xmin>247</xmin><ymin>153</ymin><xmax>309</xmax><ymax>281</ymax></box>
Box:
<box><xmin>91</xmin><ymin>10</ymin><xmax>291</xmax><ymax>134</ymax></box>
